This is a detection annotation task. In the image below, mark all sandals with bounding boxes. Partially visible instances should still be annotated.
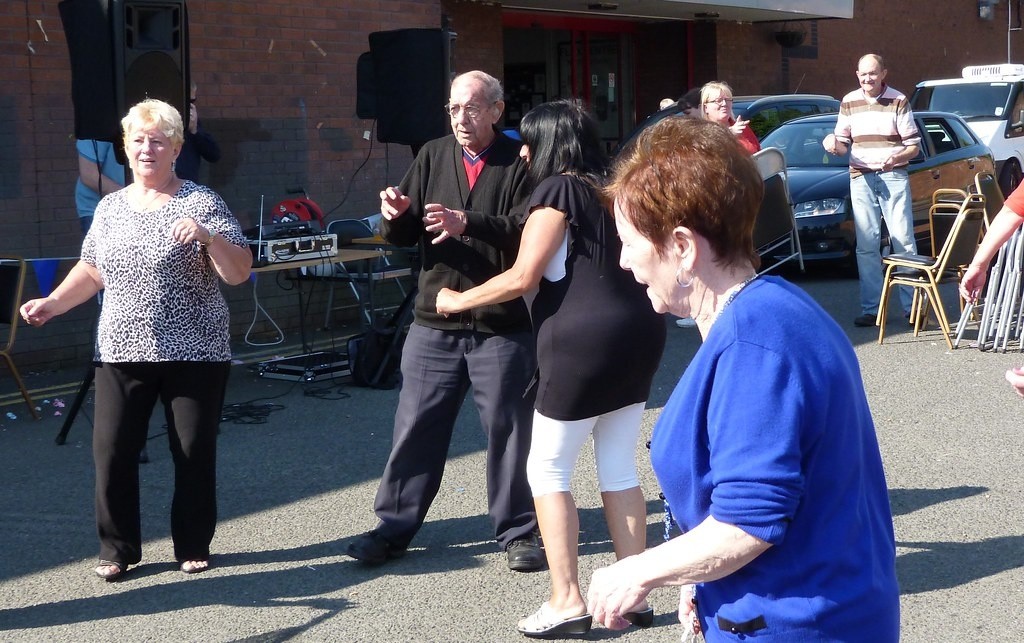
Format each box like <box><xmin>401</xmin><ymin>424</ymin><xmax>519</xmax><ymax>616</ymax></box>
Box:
<box><xmin>179</xmin><ymin>557</ymin><xmax>208</xmax><ymax>572</ymax></box>
<box><xmin>96</xmin><ymin>560</ymin><xmax>128</xmax><ymax>579</ymax></box>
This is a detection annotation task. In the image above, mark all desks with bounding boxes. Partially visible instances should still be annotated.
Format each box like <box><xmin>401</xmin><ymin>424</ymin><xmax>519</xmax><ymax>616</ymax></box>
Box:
<box><xmin>251</xmin><ymin>249</ymin><xmax>392</xmax><ymax>332</ymax></box>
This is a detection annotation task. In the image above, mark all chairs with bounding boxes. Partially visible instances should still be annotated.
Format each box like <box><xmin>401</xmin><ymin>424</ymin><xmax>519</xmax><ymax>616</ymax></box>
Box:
<box><xmin>0</xmin><ymin>257</ymin><xmax>43</xmax><ymax>422</ymax></box>
<box><xmin>321</xmin><ymin>214</ymin><xmax>417</xmax><ymax>335</ymax></box>
<box><xmin>54</xmin><ymin>289</ymin><xmax>147</xmax><ymax>462</ymax></box>
<box><xmin>747</xmin><ymin>146</ymin><xmax>805</xmax><ymax>281</ymax></box>
<box><xmin>875</xmin><ymin>171</ymin><xmax>1024</xmax><ymax>354</ymax></box>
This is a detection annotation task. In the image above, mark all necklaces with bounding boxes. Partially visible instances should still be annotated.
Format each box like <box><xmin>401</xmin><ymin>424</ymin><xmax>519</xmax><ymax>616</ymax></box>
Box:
<box><xmin>133</xmin><ymin>187</ymin><xmax>166</xmax><ymax>212</ymax></box>
<box><xmin>710</xmin><ymin>275</ymin><xmax>758</xmax><ymax>328</ymax></box>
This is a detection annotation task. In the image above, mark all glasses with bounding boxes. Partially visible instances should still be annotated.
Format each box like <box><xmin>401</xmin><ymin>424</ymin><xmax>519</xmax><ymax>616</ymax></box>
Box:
<box><xmin>706</xmin><ymin>97</ymin><xmax>733</xmax><ymax>105</ymax></box>
<box><xmin>190</xmin><ymin>98</ymin><xmax>197</xmax><ymax>103</ymax></box>
<box><xmin>444</xmin><ymin>101</ymin><xmax>498</xmax><ymax>118</ymax></box>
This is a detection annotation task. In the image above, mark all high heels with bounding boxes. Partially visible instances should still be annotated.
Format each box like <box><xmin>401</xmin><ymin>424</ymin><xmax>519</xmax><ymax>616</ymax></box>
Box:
<box><xmin>622</xmin><ymin>606</ymin><xmax>653</xmax><ymax>625</ymax></box>
<box><xmin>517</xmin><ymin>601</ymin><xmax>592</xmax><ymax>636</ymax></box>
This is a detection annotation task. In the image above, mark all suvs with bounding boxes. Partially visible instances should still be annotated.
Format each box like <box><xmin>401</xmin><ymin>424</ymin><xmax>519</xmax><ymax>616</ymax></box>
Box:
<box><xmin>601</xmin><ymin>92</ymin><xmax>844</xmax><ymax>177</ymax></box>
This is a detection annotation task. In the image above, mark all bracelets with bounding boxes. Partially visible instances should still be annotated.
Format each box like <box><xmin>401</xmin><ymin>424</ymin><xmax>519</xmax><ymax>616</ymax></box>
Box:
<box><xmin>204</xmin><ymin>228</ymin><xmax>216</xmax><ymax>246</ymax></box>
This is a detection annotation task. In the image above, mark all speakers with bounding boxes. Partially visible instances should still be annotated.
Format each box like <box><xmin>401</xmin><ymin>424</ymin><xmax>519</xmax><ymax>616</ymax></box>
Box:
<box><xmin>369</xmin><ymin>27</ymin><xmax>458</xmax><ymax>146</ymax></box>
<box><xmin>58</xmin><ymin>0</ymin><xmax>191</xmax><ymax>142</ymax></box>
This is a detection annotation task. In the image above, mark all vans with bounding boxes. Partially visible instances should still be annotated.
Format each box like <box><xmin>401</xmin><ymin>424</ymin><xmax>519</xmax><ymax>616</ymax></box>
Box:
<box><xmin>908</xmin><ymin>63</ymin><xmax>1024</xmax><ymax>204</ymax></box>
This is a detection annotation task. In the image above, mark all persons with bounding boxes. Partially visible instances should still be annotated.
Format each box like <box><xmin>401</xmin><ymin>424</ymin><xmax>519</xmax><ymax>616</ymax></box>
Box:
<box><xmin>18</xmin><ymin>98</ymin><xmax>253</xmax><ymax>578</ymax></box>
<box><xmin>823</xmin><ymin>53</ymin><xmax>928</xmax><ymax>329</ymax></box>
<box><xmin>175</xmin><ymin>79</ymin><xmax>221</xmax><ymax>182</ymax></box>
<box><xmin>74</xmin><ymin>139</ymin><xmax>125</xmax><ymax>317</ymax></box>
<box><xmin>959</xmin><ymin>176</ymin><xmax>1024</xmax><ymax>395</ymax></box>
<box><xmin>434</xmin><ymin>97</ymin><xmax>668</xmax><ymax>636</ymax></box>
<box><xmin>348</xmin><ymin>75</ymin><xmax>545</xmax><ymax>570</ymax></box>
<box><xmin>587</xmin><ymin>116</ymin><xmax>902</xmax><ymax>643</ymax></box>
<box><xmin>679</xmin><ymin>81</ymin><xmax>760</xmax><ymax>154</ymax></box>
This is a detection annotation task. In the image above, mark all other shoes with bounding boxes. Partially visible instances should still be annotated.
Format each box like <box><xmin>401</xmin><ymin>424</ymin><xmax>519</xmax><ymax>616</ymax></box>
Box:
<box><xmin>909</xmin><ymin>312</ymin><xmax>929</xmax><ymax>329</ymax></box>
<box><xmin>855</xmin><ymin>314</ymin><xmax>887</xmax><ymax>325</ymax></box>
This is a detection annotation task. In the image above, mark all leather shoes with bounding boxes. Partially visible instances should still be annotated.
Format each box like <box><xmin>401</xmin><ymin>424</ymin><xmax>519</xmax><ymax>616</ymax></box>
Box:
<box><xmin>506</xmin><ymin>527</ymin><xmax>546</xmax><ymax>569</ymax></box>
<box><xmin>348</xmin><ymin>531</ymin><xmax>406</xmax><ymax>563</ymax></box>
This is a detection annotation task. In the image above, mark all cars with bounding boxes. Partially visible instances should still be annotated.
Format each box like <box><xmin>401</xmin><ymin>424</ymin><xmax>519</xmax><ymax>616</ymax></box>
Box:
<box><xmin>752</xmin><ymin>111</ymin><xmax>1000</xmax><ymax>280</ymax></box>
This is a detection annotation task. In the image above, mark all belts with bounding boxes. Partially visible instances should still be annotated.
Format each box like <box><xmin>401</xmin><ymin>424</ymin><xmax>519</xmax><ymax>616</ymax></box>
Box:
<box><xmin>850</xmin><ymin>163</ymin><xmax>910</xmax><ymax>179</ymax></box>
